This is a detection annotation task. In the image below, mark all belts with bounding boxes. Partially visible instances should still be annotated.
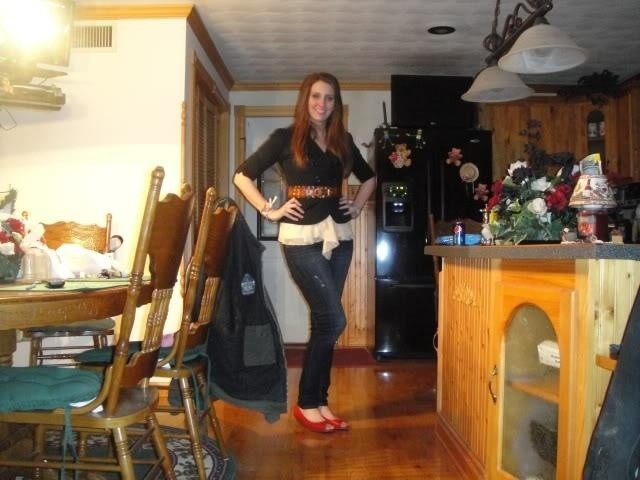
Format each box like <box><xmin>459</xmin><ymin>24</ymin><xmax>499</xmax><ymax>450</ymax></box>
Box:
<box><xmin>287</xmin><ymin>185</ymin><xmax>339</xmax><ymax>200</ymax></box>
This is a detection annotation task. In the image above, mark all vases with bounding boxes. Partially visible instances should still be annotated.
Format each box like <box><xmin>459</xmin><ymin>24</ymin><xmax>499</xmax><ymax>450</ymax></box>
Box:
<box><xmin>1</xmin><ymin>254</ymin><xmax>19</xmax><ymax>284</ymax></box>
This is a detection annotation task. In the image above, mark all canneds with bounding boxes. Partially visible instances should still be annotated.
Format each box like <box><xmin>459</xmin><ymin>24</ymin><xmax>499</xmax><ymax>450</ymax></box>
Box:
<box><xmin>453</xmin><ymin>221</ymin><xmax>465</xmax><ymax>247</ymax></box>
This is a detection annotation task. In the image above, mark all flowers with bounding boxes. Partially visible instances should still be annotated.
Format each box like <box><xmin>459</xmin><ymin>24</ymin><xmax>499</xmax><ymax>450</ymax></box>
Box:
<box><xmin>478</xmin><ymin>118</ymin><xmax>624</xmax><ymax>245</ymax></box>
<box><xmin>0</xmin><ymin>212</ymin><xmax>52</xmax><ymax>259</ymax></box>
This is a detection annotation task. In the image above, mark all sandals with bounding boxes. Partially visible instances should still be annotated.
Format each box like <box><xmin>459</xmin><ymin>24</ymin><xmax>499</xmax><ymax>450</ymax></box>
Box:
<box><xmin>322</xmin><ymin>412</ymin><xmax>349</xmax><ymax>430</ymax></box>
<box><xmin>294</xmin><ymin>404</ymin><xmax>335</xmax><ymax>432</ymax></box>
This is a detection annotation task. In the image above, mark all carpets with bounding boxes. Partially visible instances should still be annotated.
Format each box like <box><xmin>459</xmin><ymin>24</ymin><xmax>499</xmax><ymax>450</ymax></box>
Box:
<box><xmin>15</xmin><ymin>423</ymin><xmax>239</xmax><ymax>480</ymax></box>
<box><xmin>284</xmin><ymin>347</ymin><xmax>377</xmax><ymax>369</ymax></box>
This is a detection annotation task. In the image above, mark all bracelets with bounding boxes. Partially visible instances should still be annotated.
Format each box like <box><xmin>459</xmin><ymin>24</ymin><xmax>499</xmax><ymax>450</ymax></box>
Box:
<box><xmin>260</xmin><ymin>195</ymin><xmax>277</xmax><ymax>217</ymax></box>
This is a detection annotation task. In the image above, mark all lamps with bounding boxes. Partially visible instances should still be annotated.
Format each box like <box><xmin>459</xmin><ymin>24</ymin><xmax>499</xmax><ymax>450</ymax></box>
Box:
<box><xmin>461</xmin><ymin>0</ymin><xmax>590</xmax><ymax>104</ymax></box>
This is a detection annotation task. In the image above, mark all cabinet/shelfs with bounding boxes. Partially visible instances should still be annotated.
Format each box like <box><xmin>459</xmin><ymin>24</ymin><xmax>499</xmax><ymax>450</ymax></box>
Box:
<box><xmin>424</xmin><ymin>244</ymin><xmax>639</xmax><ymax>480</ymax></box>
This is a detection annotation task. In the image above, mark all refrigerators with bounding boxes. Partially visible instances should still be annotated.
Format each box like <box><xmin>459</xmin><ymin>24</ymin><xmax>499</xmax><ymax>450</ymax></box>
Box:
<box><xmin>375</xmin><ymin>125</ymin><xmax>493</xmax><ymax>361</ymax></box>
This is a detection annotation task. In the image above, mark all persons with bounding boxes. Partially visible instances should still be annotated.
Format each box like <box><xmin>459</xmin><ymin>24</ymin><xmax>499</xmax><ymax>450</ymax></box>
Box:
<box><xmin>232</xmin><ymin>71</ymin><xmax>380</xmax><ymax>434</ymax></box>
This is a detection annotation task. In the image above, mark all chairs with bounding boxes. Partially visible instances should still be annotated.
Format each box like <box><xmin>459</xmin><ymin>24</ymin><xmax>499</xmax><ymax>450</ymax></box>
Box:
<box><xmin>0</xmin><ymin>165</ymin><xmax>197</xmax><ymax>480</ymax></box>
<box><xmin>24</xmin><ymin>211</ymin><xmax>117</xmax><ymax>368</ymax></box>
<box><xmin>61</xmin><ymin>184</ymin><xmax>240</xmax><ymax>480</ymax></box>
